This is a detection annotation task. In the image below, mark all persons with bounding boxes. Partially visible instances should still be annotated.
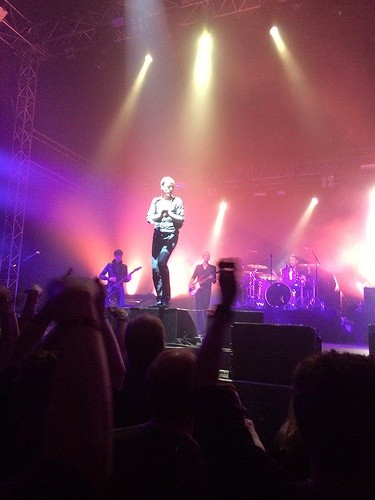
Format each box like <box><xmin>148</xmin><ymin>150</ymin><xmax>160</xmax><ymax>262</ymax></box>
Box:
<box><xmin>146</xmin><ymin>175</ymin><xmax>185</xmax><ymax>310</ymax></box>
<box><xmin>281</xmin><ymin>254</ymin><xmax>310</xmax><ymax>298</ymax></box>
<box><xmin>187</xmin><ymin>251</ymin><xmax>217</xmax><ymax>338</ymax></box>
<box><xmin>1</xmin><ymin>275</ymin><xmax>375</xmax><ymax>500</ymax></box>
<box><xmin>99</xmin><ymin>250</ymin><xmax>132</xmax><ymax>308</ymax></box>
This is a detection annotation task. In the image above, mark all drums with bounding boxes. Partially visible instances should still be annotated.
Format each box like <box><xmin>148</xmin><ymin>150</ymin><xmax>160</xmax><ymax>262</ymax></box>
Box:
<box><xmin>291</xmin><ymin>284</ymin><xmax>313</xmax><ymax>306</ymax></box>
<box><xmin>244</xmin><ymin>278</ymin><xmax>261</xmax><ymax>300</ymax></box>
<box><xmin>264</xmin><ymin>281</ymin><xmax>293</xmax><ymax>308</ymax></box>
<box><xmin>280</xmin><ymin>267</ymin><xmax>297</xmax><ymax>285</ymax></box>
<box><xmin>297</xmin><ymin>273</ymin><xmax>315</xmax><ymax>289</ymax></box>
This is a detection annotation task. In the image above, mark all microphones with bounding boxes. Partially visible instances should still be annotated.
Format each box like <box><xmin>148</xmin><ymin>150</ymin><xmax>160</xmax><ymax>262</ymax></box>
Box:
<box><xmin>31</xmin><ymin>251</ymin><xmax>39</xmax><ymax>258</ymax></box>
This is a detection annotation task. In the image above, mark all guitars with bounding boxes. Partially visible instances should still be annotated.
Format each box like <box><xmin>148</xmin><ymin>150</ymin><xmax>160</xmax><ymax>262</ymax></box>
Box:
<box><xmin>188</xmin><ymin>270</ymin><xmax>219</xmax><ymax>297</ymax></box>
<box><xmin>103</xmin><ymin>266</ymin><xmax>142</xmax><ymax>301</ymax></box>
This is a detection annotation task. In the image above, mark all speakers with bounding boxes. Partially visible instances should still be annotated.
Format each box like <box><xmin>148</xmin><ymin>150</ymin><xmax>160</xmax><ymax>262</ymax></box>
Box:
<box><xmin>229</xmin><ymin>321</ymin><xmax>322</xmax><ymax>386</ymax></box>
<box><xmin>127</xmin><ymin>307</ymin><xmax>202</xmax><ymax>346</ymax></box>
<box><xmin>208</xmin><ymin>308</ymin><xmax>265</xmax><ymax>349</ymax></box>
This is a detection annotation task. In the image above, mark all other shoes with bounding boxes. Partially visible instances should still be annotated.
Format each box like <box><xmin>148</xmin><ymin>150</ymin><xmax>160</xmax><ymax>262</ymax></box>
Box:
<box><xmin>148</xmin><ymin>300</ymin><xmax>171</xmax><ymax>309</ymax></box>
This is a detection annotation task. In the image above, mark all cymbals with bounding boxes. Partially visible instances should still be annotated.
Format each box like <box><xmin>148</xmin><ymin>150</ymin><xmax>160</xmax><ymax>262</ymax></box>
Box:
<box><xmin>247</xmin><ymin>264</ymin><xmax>269</xmax><ymax>270</ymax></box>
<box><xmin>259</xmin><ymin>274</ymin><xmax>277</xmax><ymax>281</ymax></box>
<box><xmin>244</xmin><ymin>271</ymin><xmax>262</xmax><ymax>274</ymax></box>
<box><xmin>298</xmin><ymin>263</ymin><xmax>320</xmax><ymax>268</ymax></box>
